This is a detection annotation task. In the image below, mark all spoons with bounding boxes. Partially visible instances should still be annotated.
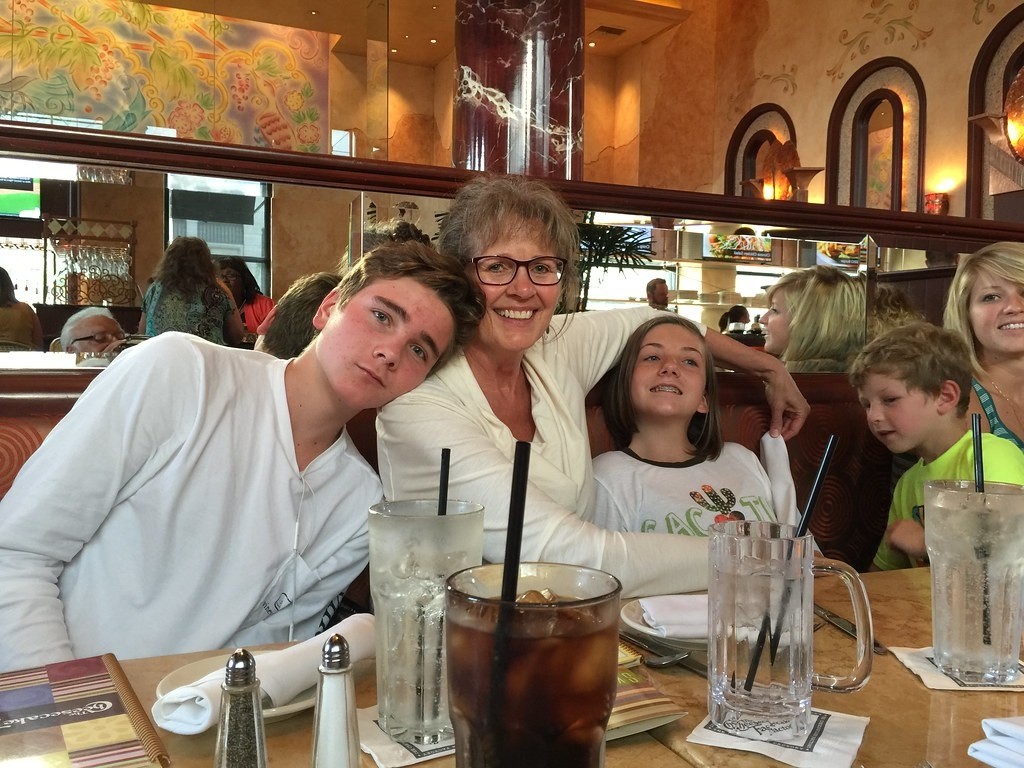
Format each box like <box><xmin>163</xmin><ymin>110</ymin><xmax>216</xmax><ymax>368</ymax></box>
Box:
<box><xmin>618</xmin><ymin>638</ymin><xmax>693</xmax><ymax>668</ymax></box>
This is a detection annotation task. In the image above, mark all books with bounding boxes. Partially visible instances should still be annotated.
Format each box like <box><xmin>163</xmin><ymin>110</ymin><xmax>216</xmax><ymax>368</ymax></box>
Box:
<box><xmin>0</xmin><ymin>653</ymin><xmax>171</xmax><ymax>768</ymax></box>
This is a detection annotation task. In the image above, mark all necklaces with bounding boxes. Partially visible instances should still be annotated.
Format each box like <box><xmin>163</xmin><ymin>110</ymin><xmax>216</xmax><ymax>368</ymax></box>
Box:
<box><xmin>989</xmin><ymin>378</ymin><xmax>1024</xmax><ymax>413</ymax></box>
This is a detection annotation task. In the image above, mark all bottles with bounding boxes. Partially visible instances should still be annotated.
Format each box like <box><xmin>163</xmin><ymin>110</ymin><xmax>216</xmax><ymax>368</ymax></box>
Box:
<box><xmin>311</xmin><ymin>631</ymin><xmax>362</xmax><ymax>768</ymax></box>
<box><xmin>213</xmin><ymin>650</ymin><xmax>269</xmax><ymax>768</ymax></box>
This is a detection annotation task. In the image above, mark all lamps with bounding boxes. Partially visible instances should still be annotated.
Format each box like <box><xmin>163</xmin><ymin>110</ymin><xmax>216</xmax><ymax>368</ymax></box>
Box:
<box><xmin>739</xmin><ymin>139</ymin><xmax>825</xmax><ymax>202</ymax></box>
<box><xmin>969</xmin><ymin>65</ymin><xmax>1024</xmax><ymax>189</ymax></box>
<box><xmin>926</xmin><ymin>191</ymin><xmax>950</xmax><ymax>217</ymax></box>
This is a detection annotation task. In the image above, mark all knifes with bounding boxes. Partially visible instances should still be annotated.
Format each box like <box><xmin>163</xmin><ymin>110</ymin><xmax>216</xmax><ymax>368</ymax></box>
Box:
<box><xmin>617</xmin><ymin>628</ymin><xmax>708</xmax><ymax>677</ymax></box>
<box><xmin>814</xmin><ymin>602</ymin><xmax>888</xmax><ymax>655</ymax></box>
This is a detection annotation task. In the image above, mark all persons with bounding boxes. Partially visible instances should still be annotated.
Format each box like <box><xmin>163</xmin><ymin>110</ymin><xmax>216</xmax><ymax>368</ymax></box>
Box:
<box><xmin>941</xmin><ymin>243</ymin><xmax>1024</xmax><ymax>448</ymax></box>
<box><xmin>851</xmin><ymin>324</ymin><xmax>1024</xmax><ymax>573</ymax></box>
<box><xmin>376</xmin><ymin>175</ymin><xmax>810</xmax><ymax>605</ymax></box>
<box><xmin>0</xmin><ymin>237</ymin><xmax>494</xmax><ymax>677</ymax></box>
<box><xmin>589</xmin><ymin>316</ymin><xmax>823</xmax><ymax>558</ymax></box>
<box><xmin>0</xmin><ymin>219</ymin><xmax>926</xmax><ymax>371</ymax></box>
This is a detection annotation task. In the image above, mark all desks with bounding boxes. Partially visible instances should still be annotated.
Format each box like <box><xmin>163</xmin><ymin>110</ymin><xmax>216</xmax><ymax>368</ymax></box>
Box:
<box><xmin>619</xmin><ymin>564</ymin><xmax>1024</xmax><ymax>768</ymax></box>
<box><xmin>118</xmin><ymin>640</ymin><xmax>697</xmax><ymax>768</ymax></box>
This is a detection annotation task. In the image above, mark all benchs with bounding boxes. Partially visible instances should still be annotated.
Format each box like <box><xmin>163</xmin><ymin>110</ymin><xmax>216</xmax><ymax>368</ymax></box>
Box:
<box><xmin>1</xmin><ymin>350</ymin><xmax>921</xmax><ymax>572</ymax></box>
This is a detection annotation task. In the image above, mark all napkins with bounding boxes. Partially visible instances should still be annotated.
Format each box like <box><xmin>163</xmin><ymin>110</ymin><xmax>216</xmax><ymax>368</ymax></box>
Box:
<box><xmin>638</xmin><ymin>595</ymin><xmax>803</xmax><ymax>644</ymax></box>
<box><xmin>357</xmin><ymin>704</ymin><xmax>458</xmax><ymax>768</ymax></box>
<box><xmin>967</xmin><ymin>715</ymin><xmax>1024</xmax><ymax>768</ymax></box>
<box><xmin>149</xmin><ymin>612</ymin><xmax>381</xmax><ymax>735</ymax></box>
<box><xmin>686</xmin><ymin>697</ymin><xmax>871</xmax><ymax>768</ymax></box>
<box><xmin>886</xmin><ymin>646</ymin><xmax>1024</xmax><ymax>693</ymax></box>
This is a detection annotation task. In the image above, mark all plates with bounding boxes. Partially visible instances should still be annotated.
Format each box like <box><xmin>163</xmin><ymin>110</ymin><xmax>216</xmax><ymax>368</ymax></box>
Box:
<box><xmin>620</xmin><ymin>594</ymin><xmax>802</xmax><ymax>653</ymax></box>
<box><xmin>155</xmin><ymin>650</ymin><xmax>316</xmax><ymax>726</ymax></box>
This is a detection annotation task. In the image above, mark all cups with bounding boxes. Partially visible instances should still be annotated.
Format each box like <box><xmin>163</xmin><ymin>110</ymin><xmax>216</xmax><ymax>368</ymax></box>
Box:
<box><xmin>445</xmin><ymin>561</ymin><xmax>623</xmax><ymax>768</ymax></box>
<box><xmin>708</xmin><ymin>520</ymin><xmax>874</xmax><ymax>741</ymax></box>
<box><xmin>368</xmin><ymin>500</ymin><xmax>485</xmax><ymax>744</ymax></box>
<box><xmin>924</xmin><ymin>480</ymin><xmax>1024</xmax><ymax>683</ymax></box>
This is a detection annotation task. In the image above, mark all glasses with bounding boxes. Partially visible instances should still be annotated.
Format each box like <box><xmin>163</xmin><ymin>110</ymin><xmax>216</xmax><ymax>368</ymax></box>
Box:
<box><xmin>468</xmin><ymin>254</ymin><xmax>569</xmax><ymax>287</ymax></box>
<box><xmin>215</xmin><ymin>273</ymin><xmax>244</xmax><ymax>281</ymax></box>
<box><xmin>70</xmin><ymin>330</ymin><xmax>129</xmax><ymax>346</ymax></box>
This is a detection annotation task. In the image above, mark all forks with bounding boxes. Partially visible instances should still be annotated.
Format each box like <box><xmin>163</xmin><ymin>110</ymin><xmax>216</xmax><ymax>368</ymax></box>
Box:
<box><xmin>813</xmin><ymin>621</ymin><xmax>827</xmax><ymax>633</ymax></box>
